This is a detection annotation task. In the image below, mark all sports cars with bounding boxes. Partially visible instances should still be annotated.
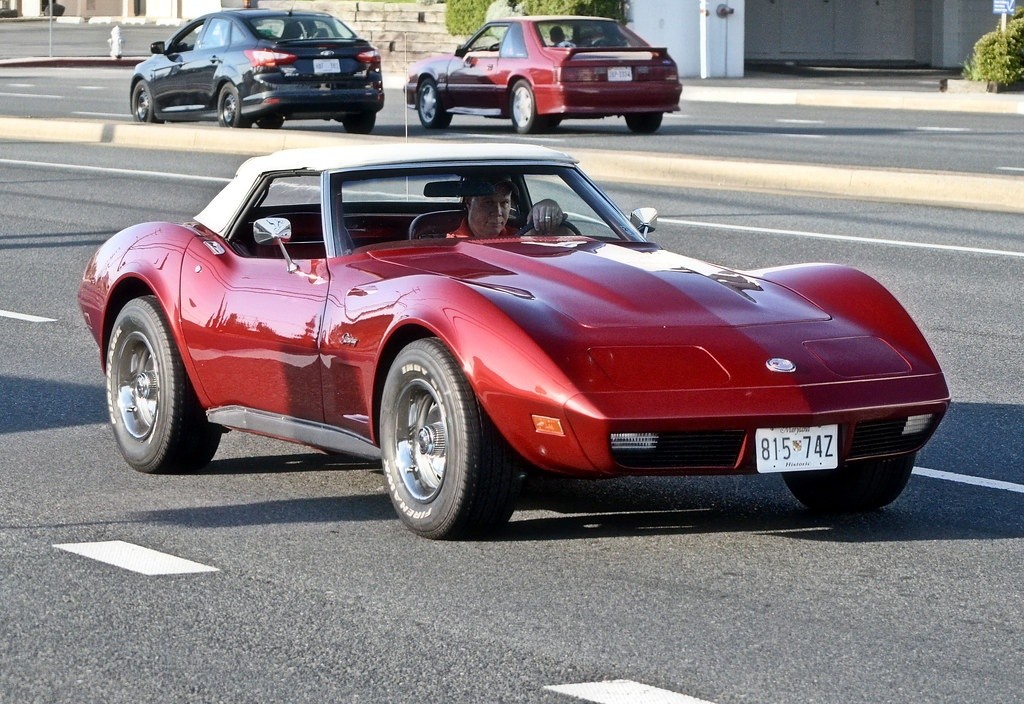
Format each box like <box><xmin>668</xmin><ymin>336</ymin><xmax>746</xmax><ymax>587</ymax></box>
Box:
<box><xmin>77</xmin><ymin>147</ymin><xmax>952</xmax><ymax>541</ymax></box>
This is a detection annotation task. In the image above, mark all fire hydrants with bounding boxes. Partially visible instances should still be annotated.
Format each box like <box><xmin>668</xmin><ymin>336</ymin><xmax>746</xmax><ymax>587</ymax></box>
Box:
<box><xmin>106</xmin><ymin>25</ymin><xmax>125</xmax><ymax>58</ymax></box>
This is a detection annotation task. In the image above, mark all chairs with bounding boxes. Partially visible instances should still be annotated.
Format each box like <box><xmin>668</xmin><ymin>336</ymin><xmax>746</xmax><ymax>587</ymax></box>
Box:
<box><xmin>409</xmin><ymin>209</ymin><xmax>463</xmax><ymax>240</ymax></box>
<box><xmin>253</xmin><ymin>212</ymin><xmax>356</xmax><ymax>259</ymax></box>
<box><xmin>223</xmin><ymin>22</ymin><xmax>327</xmax><ymax>45</ymax></box>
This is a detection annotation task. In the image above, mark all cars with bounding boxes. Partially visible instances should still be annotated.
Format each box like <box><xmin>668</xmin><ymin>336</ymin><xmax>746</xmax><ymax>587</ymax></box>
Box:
<box><xmin>404</xmin><ymin>16</ymin><xmax>683</xmax><ymax>137</ymax></box>
<box><xmin>128</xmin><ymin>4</ymin><xmax>385</xmax><ymax>134</ymax></box>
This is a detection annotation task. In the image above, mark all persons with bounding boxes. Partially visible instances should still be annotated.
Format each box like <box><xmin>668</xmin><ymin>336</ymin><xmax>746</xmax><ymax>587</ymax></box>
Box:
<box><xmin>550</xmin><ymin>25</ymin><xmax>566</xmax><ymax>47</ymax></box>
<box><xmin>446</xmin><ymin>174</ymin><xmax>573</xmax><ymax>236</ymax></box>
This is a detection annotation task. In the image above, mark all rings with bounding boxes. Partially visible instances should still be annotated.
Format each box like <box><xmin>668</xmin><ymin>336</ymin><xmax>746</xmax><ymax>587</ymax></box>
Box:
<box><xmin>545</xmin><ymin>216</ymin><xmax>551</xmax><ymax>219</ymax></box>
